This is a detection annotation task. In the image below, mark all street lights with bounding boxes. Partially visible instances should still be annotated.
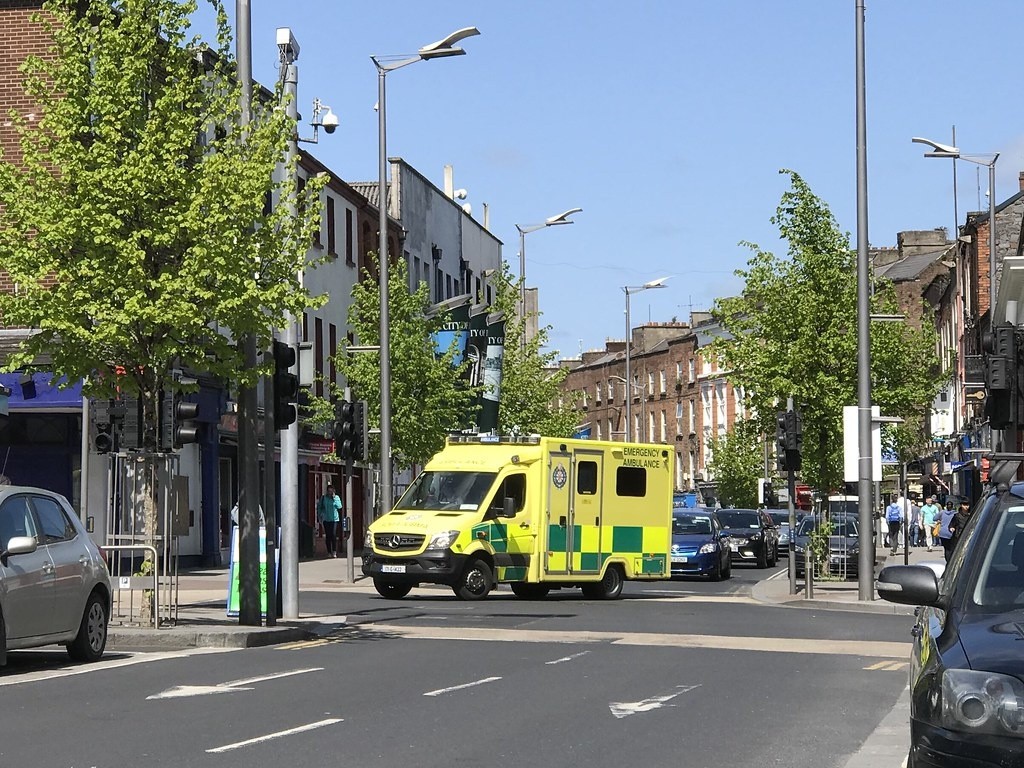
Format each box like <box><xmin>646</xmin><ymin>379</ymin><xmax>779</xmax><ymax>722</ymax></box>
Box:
<box><xmin>372</xmin><ymin>25</ymin><xmax>483</xmax><ymax>512</ymax></box>
<box><xmin>624</xmin><ymin>274</ymin><xmax>676</xmax><ymax>442</ymax></box>
<box><xmin>912</xmin><ymin>137</ymin><xmax>1000</xmax><ymax>332</ymax></box>
<box><xmin>608</xmin><ymin>374</ymin><xmax>650</xmax><ymax>441</ymax></box>
<box><xmin>515</xmin><ymin>207</ymin><xmax>582</xmax><ymax>370</ymax></box>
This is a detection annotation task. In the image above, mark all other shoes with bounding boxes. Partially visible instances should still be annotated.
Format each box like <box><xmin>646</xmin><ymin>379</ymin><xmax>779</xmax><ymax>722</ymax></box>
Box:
<box><xmin>333</xmin><ymin>551</ymin><xmax>337</xmax><ymax>557</ymax></box>
<box><xmin>328</xmin><ymin>551</ymin><xmax>332</xmax><ymax>558</ymax></box>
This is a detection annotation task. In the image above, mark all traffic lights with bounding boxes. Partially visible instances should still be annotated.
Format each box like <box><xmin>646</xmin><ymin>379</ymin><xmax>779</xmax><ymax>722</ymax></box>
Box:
<box><xmin>976</xmin><ymin>320</ymin><xmax>1020</xmax><ymax>427</ymax></box>
<box><xmin>778</xmin><ymin>409</ymin><xmax>798</xmax><ymax>452</ymax></box>
<box><xmin>332</xmin><ymin>398</ymin><xmax>357</xmax><ymax>460</ymax></box>
<box><xmin>272</xmin><ymin>339</ymin><xmax>301</xmax><ymax>430</ymax></box>
<box><xmin>171</xmin><ymin>369</ymin><xmax>200</xmax><ymax>455</ymax></box>
<box><xmin>93</xmin><ymin>420</ymin><xmax>112</xmax><ymax>453</ymax></box>
<box><xmin>778</xmin><ymin>452</ymin><xmax>801</xmax><ymax>472</ymax></box>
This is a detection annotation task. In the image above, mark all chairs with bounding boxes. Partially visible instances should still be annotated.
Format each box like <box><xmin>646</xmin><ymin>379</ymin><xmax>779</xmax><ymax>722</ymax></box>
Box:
<box><xmin>1001</xmin><ymin>531</ymin><xmax>1024</xmax><ymax>603</ymax></box>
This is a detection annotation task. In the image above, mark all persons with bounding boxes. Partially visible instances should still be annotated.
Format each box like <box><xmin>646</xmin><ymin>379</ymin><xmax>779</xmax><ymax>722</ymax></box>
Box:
<box><xmin>317</xmin><ymin>485</ymin><xmax>343</xmax><ymax>559</ymax></box>
<box><xmin>948</xmin><ymin>497</ymin><xmax>972</xmax><ymax>543</ymax></box>
<box><xmin>897</xmin><ymin>489</ymin><xmax>943</xmax><ymax>546</ymax></box>
<box><xmin>884</xmin><ymin>497</ymin><xmax>904</xmax><ymax>556</ymax></box>
<box><xmin>919</xmin><ymin>498</ymin><xmax>939</xmax><ymax>552</ymax></box>
<box><xmin>932</xmin><ymin>501</ymin><xmax>958</xmax><ymax>563</ymax></box>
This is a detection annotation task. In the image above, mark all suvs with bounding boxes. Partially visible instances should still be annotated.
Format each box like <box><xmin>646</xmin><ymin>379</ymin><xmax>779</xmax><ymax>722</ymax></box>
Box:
<box><xmin>713</xmin><ymin>507</ymin><xmax>781</xmax><ymax>570</ymax></box>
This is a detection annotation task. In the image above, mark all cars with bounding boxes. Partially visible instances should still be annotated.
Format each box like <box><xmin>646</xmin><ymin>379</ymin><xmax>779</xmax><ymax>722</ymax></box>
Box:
<box><xmin>670</xmin><ymin>508</ymin><xmax>733</xmax><ymax>581</ymax></box>
<box><xmin>873</xmin><ymin>450</ymin><xmax>1024</xmax><ymax>768</ymax></box>
<box><xmin>765</xmin><ymin>509</ymin><xmax>806</xmax><ymax>554</ymax></box>
<box><xmin>0</xmin><ymin>481</ymin><xmax>117</xmax><ymax>663</ymax></box>
<box><xmin>788</xmin><ymin>495</ymin><xmax>881</xmax><ymax>584</ymax></box>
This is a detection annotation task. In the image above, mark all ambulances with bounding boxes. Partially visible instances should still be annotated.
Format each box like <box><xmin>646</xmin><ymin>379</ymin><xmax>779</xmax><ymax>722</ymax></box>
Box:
<box><xmin>360</xmin><ymin>425</ymin><xmax>674</xmax><ymax>603</ymax></box>
